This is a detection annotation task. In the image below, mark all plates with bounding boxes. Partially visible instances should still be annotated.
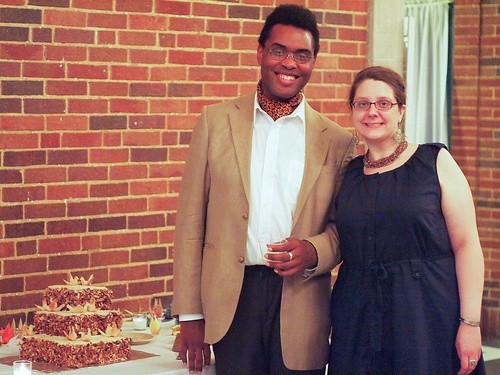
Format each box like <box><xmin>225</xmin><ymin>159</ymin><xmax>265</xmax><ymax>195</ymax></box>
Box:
<box><xmin>123</xmin><ymin>331</ymin><xmax>156</xmax><ymax>345</ymax></box>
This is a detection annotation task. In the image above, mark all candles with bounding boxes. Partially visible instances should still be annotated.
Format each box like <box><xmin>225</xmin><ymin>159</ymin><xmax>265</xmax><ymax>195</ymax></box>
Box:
<box><xmin>133</xmin><ymin>315</ymin><xmax>147</xmax><ymax>330</ymax></box>
<box><xmin>13</xmin><ymin>360</ymin><xmax>33</xmax><ymax>375</ymax></box>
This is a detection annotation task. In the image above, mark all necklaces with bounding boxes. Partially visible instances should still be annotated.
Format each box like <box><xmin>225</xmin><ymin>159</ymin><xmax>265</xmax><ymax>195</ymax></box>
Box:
<box><xmin>362</xmin><ymin>138</ymin><xmax>408</xmax><ymax>168</ymax></box>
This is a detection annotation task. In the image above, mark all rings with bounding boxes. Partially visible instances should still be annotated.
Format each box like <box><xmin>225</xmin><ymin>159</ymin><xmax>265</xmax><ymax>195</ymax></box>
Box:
<box><xmin>468</xmin><ymin>359</ymin><xmax>476</xmax><ymax>365</ymax></box>
<box><xmin>289</xmin><ymin>252</ymin><xmax>293</xmax><ymax>261</ymax></box>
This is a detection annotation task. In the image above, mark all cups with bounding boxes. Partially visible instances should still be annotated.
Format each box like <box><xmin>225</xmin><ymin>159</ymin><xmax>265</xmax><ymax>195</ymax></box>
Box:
<box><xmin>13</xmin><ymin>360</ymin><xmax>32</xmax><ymax>375</ymax></box>
<box><xmin>133</xmin><ymin>313</ymin><xmax>147</xmax><ymax>331</ymax></box>
<box><xmin>257</xmin><ymin>231</ymin><xmax>288</xmax><ymax>265</ymax></box>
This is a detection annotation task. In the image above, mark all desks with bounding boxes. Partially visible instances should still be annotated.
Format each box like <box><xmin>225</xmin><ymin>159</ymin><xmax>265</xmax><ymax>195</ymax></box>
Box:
<box><xmin>0</xmin><ymin>308</ymin><xmax>217</xmax><ymax>375</ymax></box>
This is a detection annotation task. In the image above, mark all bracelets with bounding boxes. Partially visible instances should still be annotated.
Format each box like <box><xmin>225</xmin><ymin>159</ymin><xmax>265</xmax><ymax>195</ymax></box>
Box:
<box><xmin>460</xmin><ymin>318</ymin><xmax>481</xmax><ymax>327</ymax></box>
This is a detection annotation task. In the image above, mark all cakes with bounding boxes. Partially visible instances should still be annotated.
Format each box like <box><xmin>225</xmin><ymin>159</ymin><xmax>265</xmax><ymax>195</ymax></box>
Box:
<box><xmin>19</xmin><ymin>273</ymin><xmax>131</xmax><ymax>368</ymax></box>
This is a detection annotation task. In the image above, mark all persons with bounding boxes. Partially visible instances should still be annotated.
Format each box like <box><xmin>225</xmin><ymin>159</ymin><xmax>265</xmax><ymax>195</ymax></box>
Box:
<box><xmin>328</xmin><ymin>66</ymin><xmax>484</xmax><ymax>375</ymax></box>
<box><xmin>169</xmin><ymin>2</ymin><xmax>354</xmax><ymax>375</ymax></box>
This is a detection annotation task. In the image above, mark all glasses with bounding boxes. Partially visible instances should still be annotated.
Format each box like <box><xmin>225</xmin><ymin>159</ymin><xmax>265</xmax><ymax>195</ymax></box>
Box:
<box><xmin>349</xmin><ymin>100</ymin><xmax>402</xmax><ymax>111</ymax></box>
<box><xmin>259</xmin><ymin>40</ymin><xmax>315</xmax><ymax>64</ymax></box>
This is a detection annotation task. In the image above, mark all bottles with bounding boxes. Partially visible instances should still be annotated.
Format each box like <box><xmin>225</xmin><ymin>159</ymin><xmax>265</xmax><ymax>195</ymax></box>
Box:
<box><xmin>149</xmin><ymin>313</ymin><xmax>160</xmax><ymax>335</ymax></box>
